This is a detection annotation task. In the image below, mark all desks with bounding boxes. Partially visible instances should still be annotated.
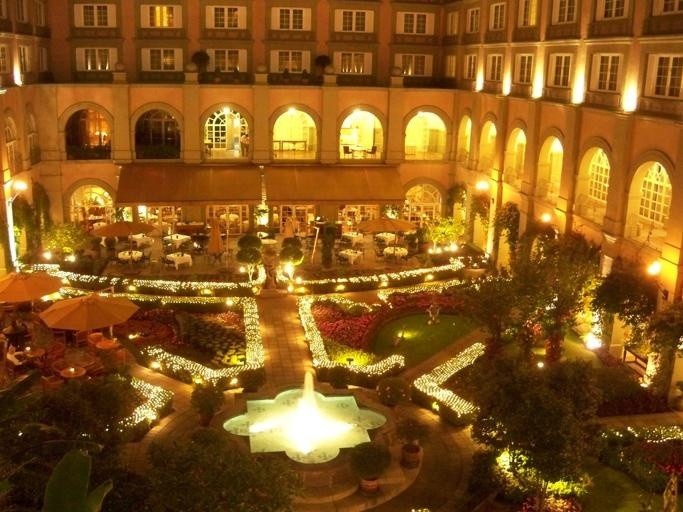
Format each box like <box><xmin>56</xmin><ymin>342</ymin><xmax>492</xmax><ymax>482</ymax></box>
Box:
<box><xmin>166</xmin><ymin>251</ymin><xmax>193</xmax><ymax>270</ymax></box>
<box><xmin>128</xmin><ymin>233</ymin><xmax>152</xmax><ymax>248</ymax></box>
<box><xmin>337</xmin><ymin>248</ymin><xmax>362</xmax><ymax>266</ymax></box>
<box><xmin>342</xmin><ymin>230</ymin><xmax>366</xmax><ymax>245</ymax></box>
<box><xmin>119</xmin><ymin>250</ymin><xmax>142</xmax><ymax>275</ymax></box>
<box><xmin>375</xmin><ymin>231</ymin><xmax>398</xmax><ymax>245</ymax></box>
<box><xmin>383</xmin><ymin>246</ymin><xmax>408</xmax><ymax>266</ymax></box>
<box><xmin>25</xmin><ymin>346</ymin><xmax>47</xmax><ymax>364</ymax></box>
<box><xmin>95</xmin><ymin>338</ymin><xmax>121</xmax><ymax>356</ymax></box>
<box><xmin>349</xmin><ymin>145</ymin><xmax>368</xmax><ymax>158</ymax></box>
<box><xmin>163</xmin><ymin>234</ymin><xmax>191</xmax><ymax>249</ymax></box>
<box><xmin>61</xmin><ymin>365</ymin><xmax>87</xmax><ymax>380</ymax></box>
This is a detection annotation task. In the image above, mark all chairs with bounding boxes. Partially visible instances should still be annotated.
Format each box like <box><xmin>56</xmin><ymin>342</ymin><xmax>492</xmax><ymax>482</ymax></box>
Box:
<box><xmin>342</xmin><ymin>145</ymin><xmax>352</xmax><ymax>158</ymax></box>
<box><xmin>367</xmin><ymin>146</ymin><xmax>377</xmax><ymax>159</ymax></box>
<box><xmin>7</xmin><ymin>359</ymin><xmax>26</xmax><ymax>376</ymax></box>
